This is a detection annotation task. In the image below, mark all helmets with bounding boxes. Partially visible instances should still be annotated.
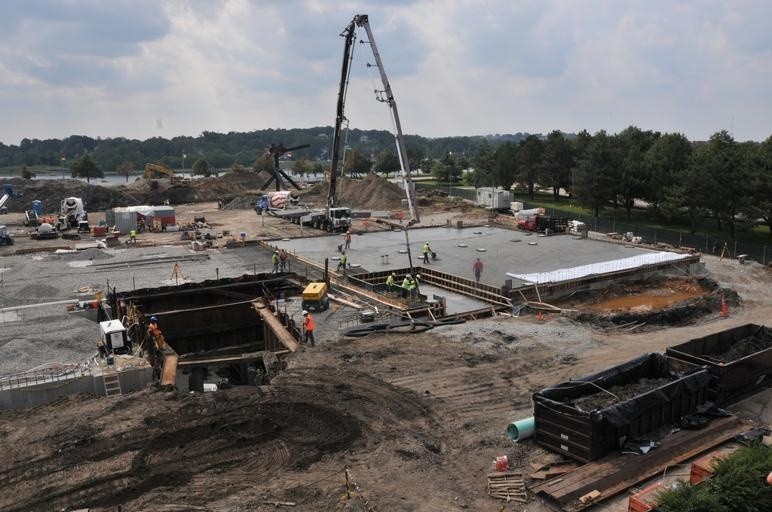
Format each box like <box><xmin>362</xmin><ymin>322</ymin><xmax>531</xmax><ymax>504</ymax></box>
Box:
<box><xmin>416</xmin><ymin>274</ymin><xmax>421</xmax><ymax>278</ymax></box>
<box><xmin>151</xmin><ymin>317</ymin><xmax>158</xmax><ymax>321</ymax></box>
<box><xmin>301</xmin><ymin>310</ymin><xmax>308</xmax><ymax>315</ymax></box>
<box><xmin>392</xmin><ymin>272</ymin><xmax>397</xmax><ymax>277</ymax></box>
<box><xmin>406</xmin><ymin>274</ymin><xmax>412</xmax><ymax>278</ymax></box>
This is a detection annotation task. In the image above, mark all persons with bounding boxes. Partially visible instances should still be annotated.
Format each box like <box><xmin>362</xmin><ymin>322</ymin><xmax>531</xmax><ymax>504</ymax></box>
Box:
<box><xmin>302</xmin><ymin>310</ymin><xmax>316</xmax><ymax>347</ymax></box>
<box><xmin>343</xmin><ymin>230</ymin><xmax>352</xmax><ymax>249</ymax></box>
<box><xmin>472</xmin><ymin>258</ymin><xmax>484</xmax><ymax>282</ymax></box>
<box><xmin>385</xmin><ymin>272</ymin><xmax>421</xmax><ymax>304</ymax></box>
<box><xmin>129</xmin><ymin>227</ymin><xmax>137</xmax><ymax>247</ymax></box>
<box><xmin>149</xmin><ymin>315</ymin><xmax>168</xmax><ymax>350</ymax></box>
<box><xmin>272</xmin><ymin>249</ymin><xmax>287</xmax><ymax>273</ymax></box>
<box><xmin>422</xmin><ymin>241</ymin><xmax>432</xmax><ymax>264</ymax></box>
<box><xmin>335</xmin><ymin>250</ymin><xmax>347</xmax><ymax>271</ymax></box>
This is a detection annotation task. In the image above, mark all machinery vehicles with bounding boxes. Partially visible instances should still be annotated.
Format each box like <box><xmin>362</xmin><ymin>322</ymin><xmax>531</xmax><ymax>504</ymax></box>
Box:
<box><xmin>58</xmin><ymin>195</ymin><xmax>85</xmax><ymax>228</ymax></box>
<box><xmin>286</xmin><ymin>9</ymin><xmax>428</xmax><ymax>234</ymax></box>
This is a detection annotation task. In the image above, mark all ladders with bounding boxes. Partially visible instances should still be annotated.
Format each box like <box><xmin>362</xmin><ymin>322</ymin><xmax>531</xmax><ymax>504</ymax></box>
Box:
<box><xmin>101</xmin><ymin>358</ymin><xmax>121</xmax><ymax>397</ymax></box>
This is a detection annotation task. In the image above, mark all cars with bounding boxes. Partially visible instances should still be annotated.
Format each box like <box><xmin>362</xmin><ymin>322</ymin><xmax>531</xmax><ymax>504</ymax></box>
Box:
<box><xmin>0</xmin><ymin>204</ymin><xmax>9</xmax><ymax>215</ymax></box>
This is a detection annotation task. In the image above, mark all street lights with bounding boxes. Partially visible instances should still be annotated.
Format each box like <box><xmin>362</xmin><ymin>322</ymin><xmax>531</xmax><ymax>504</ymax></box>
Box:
<box><xmin>62</xmin><ymin>157</ymin><xmax>67</xmax><ymax>182</ymax></box>
<box><xmin>183</xmin><ymin>151</ymin><xmax>187</xmax><ymax>180</ymax></box>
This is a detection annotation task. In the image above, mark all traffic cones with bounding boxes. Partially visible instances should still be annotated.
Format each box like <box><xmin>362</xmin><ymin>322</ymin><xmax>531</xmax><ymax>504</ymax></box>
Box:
<box><xmin>721</xmin><ymin>292</ymin><xmax>729</xmax><ymax>318</ymax></box>
